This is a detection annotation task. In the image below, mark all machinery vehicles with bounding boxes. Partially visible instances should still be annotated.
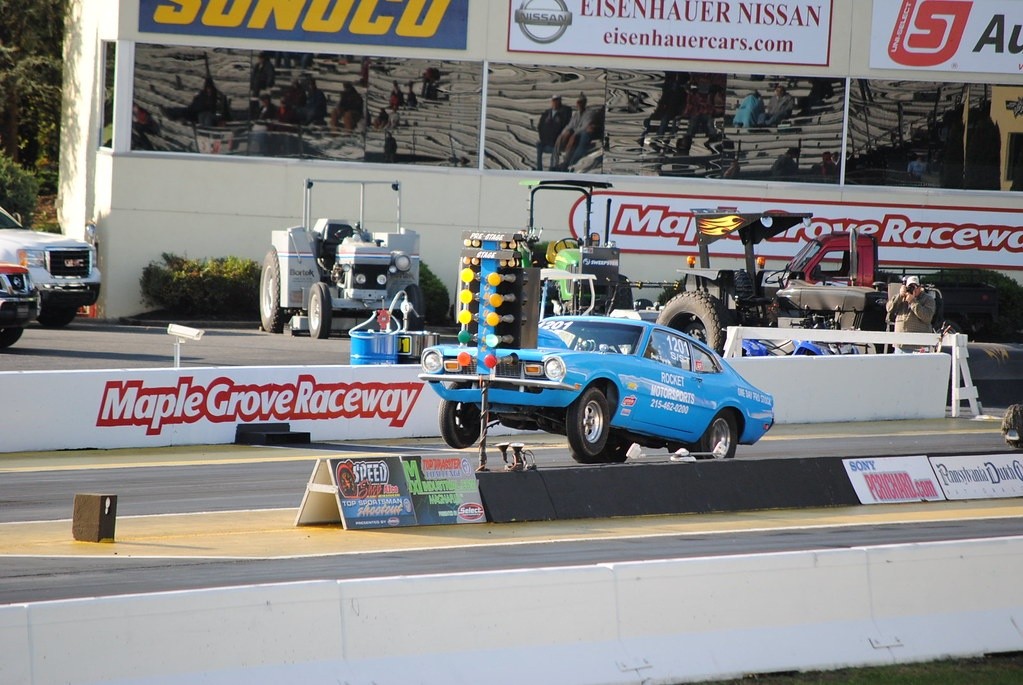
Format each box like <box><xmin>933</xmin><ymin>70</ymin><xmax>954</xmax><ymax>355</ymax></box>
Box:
<box><xmin>259</xmin><ymin>178</ymin><xmax>422</xmax><ymax>339</ymax></box>
<box><xmin>518</xmin><ymin>179</ymin><xmax>889</xmax><ymax>358</ymax></box>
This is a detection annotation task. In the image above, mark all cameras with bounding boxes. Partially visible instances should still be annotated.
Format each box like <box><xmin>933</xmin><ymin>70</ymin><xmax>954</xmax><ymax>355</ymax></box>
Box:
<box><xmin>906</xmin><ymin>283</ymin><xmax>915</xmax><ymax>294</ymax></box>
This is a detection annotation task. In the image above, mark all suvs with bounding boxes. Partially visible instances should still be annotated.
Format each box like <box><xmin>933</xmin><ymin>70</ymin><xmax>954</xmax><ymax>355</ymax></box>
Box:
<box><xmin>0</xmin><ymin>265</ymin><xmax>40</xmax><ymax>350</ymax></box>
<box><xmin>0</xmin><ymin>208</ymin><xmax>100</xmax><ymax>327</ymax></box>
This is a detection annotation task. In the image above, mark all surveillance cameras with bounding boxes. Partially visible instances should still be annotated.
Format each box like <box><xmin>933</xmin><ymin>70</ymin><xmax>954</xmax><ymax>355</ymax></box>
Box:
<box><xmin>167</xmin><ymin>323</ymin><xmax>205</xmax><ymax>340</ymax></box>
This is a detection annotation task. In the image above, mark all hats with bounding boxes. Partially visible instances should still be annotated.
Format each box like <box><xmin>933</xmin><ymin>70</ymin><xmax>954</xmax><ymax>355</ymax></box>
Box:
<box><xmin>552</xmin><ymin>94</ymin><xmax>561</xmax><ymax>100</ymax></box>
<box><xmin>906</xmin><ymin>276</ymin><xmax>919</xmax><ymax>286</ymax></box>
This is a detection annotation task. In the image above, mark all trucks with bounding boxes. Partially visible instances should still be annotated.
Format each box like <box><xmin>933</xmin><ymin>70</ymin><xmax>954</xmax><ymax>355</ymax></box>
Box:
<box><xmin>782</xmin><ymin>230</ymin><xmax>998</xmax><ymax>339</ymax></box>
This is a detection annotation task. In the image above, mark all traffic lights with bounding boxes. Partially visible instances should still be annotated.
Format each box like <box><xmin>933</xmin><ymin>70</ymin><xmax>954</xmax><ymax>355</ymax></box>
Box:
<box><xmin>457</xmin><ymin>231</ymin><xmax>541</xmax><ymax>472</ymax></box>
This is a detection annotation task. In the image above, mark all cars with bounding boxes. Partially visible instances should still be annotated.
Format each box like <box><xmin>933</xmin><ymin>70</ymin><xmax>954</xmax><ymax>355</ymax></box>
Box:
<box><xmin>419</xmin><ymin>313</ymin><xmax>776</xmax><ymax>464</ymax></box>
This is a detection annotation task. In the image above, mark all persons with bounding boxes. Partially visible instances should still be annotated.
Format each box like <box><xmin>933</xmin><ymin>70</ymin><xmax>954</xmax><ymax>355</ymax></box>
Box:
<box><xmin>372</xmin><ymin>106</ymin><xmax>399</xmax><ymax>128</ymax></box>
<box><xmin>908</xmin><ymin>158</ymin><xmax>927</xmax><ymax>176</ymax></box>
<box><xmin>772</xmin><ymin>147</ymin><xmax>801</xmax><ymax>176</ymax></box>
<box><xmin>757</xmin><ymin>87</ymin><xmax>794</xmax><ymax>127</ymax></box>
<box><xmin>383</xmin><ymin>132</ymin><xmax>398</xmax><ymax>164</ymax></box>
<box><xmin>732</xmin><ymin>88</ymin><xmax>764</xmax><ymax>129</ymax></box>
<box><xmin>249</xmin><ymin>52</ymin><xmax>327</xmax><ymax>128</ymax></box>
<box><xmin>407</xmin><ymin>81</ymin><xmax>417</xmax><ymax>107</ymax></box>
<box><xmin>885</xmin><ymin>276</ymin><xmax>936</xmax><ymax>354</ymax></box>
<box><xmin>389</xmin><ymin>80</ymin><xmax>403</xmax><ymax>111</ymax></box>
<box><xmin>421</xmin><ymin>68</ymin><xmax>441</xmax><ymax>98</ymax></box>
<box><xmin>360</xmin><ymin>62</ymin><xmax>369</xmax><ymax>83</ymax></box>
<box><xmin>198</xmin><ymin>79</ymin><xmax>217</xmax><ymax>124</ymax></box>
<box><xmin>652</xmin><ymin>72</ymin><xmax>720</xmax><ymax>144</ymax></box>
<box><xmin>928</xmin><ymin>152</ymin><xmax>944</xmax><ymax>175</ymax></box>
<box><xmin>329</xmin><ymin>81</ymin><xmax>363</xmax><ymax>131</ymax></box>
<box><xmin>820</xmin><ymin>151</ymin><xmax>838</xmax><ymax>176</ymax></box>
<box><xmin>537</xmin><ymin>90</ymin><xmax>596</xmax><ymax>172</ymax></box>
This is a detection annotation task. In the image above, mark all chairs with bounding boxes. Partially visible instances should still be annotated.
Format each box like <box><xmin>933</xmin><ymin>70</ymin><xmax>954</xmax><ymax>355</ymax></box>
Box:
<box><xmin>322</xmin><ymin>224</ymin><xmax>354</xmax><ymax>259</ymax></box>
<box><xmin>733</xmin><ymin>270</ymin><xmax>772</xmax><ymax>306</ymax></box>
<box><xmin>546</xmin><ymin>241</ymin><xmax>575</xmax><ymax>268</ymax></box>
